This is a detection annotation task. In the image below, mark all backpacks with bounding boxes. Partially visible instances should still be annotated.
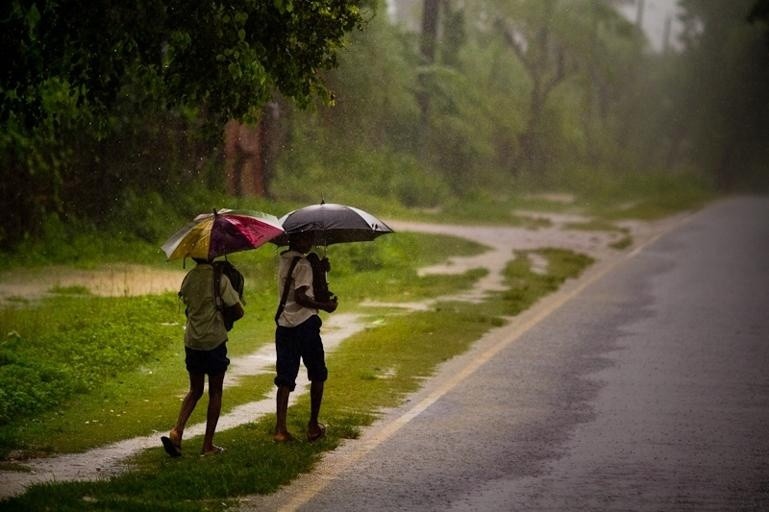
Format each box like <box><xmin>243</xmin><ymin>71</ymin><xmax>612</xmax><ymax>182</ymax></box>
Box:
<box><xmin>213</xmin><ymin>260</ymin><xmax>244</xmax><ymax>332</ymax></box>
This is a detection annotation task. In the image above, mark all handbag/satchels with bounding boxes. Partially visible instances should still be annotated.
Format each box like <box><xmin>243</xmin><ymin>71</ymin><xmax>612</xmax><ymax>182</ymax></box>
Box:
<box><xmin>306</xmin><ymin>252</ymin><xmax>334</xmax><ymax>303</ymax></box>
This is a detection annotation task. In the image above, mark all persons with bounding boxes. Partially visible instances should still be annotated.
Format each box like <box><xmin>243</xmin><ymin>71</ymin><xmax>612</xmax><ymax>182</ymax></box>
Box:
<box><xmin>272</xmin><ymin>231</ymin><xmax>339</xmax><ymax>444</ymax></box>
<box><xmin>160</xmin><ymin>245</ymin><xmax>245</xmax><ymax>458</ymax></box>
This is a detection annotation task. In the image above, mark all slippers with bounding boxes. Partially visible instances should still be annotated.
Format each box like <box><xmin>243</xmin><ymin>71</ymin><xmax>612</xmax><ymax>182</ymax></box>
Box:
<box><xmin>272</xmin><ymin>432</ymin><xmax>297</xmax><ymax>444</ymax></box>
<box><xmin>304</xmin><ymin>422</ymin><xmax>326</xmax><ymax>445</ymax></box>
<box><xmin>199</xmin><ymin>447</ymin><xmax>228</xmax><ymax>458</ymax></box>
<box><xmin>160</xmin><ymin>436</ymin><xmax>182</xmax><ymax>457</ymax></box>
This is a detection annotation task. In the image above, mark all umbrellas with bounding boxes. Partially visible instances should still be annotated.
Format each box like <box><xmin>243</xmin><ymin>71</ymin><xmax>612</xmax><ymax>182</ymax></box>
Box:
<box><xmin>158</xmin><ymin>200</ymin><xmax>286</xmax><ymax>263</ymax></box>
<box><xmin>266</xmin><ymin>189</ymin><xmax>395</xmax><ymax>286</ymax></box>
